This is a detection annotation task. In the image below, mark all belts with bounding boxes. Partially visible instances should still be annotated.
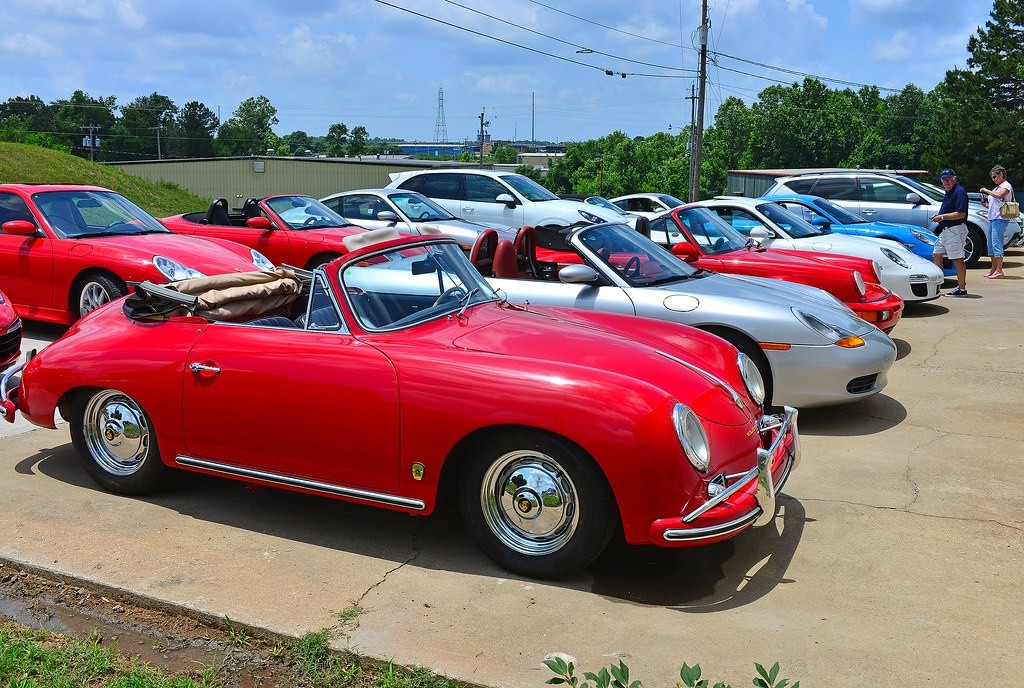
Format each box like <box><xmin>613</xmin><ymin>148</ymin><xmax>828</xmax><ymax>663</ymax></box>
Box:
<box><xmin>941</xmin><ymin>221</ymin><xmax>964</xmax><ymax>230</ymax></box>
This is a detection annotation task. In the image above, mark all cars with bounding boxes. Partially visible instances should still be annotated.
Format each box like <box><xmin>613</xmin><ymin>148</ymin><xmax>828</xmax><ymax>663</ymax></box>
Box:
<box><xmin>271</xmin><ymin>188</ymin><xmax>520</xmax><ymax>259</ymax></box>
<box><xmin>606</xmin><ymin>167</ymin><xmax>1023</xmax><ymax>304</ymax></box>
<box><xmin>0</xmin><ymin>178</ymin><xmax>280</xmax><ymax>327</ymax></box>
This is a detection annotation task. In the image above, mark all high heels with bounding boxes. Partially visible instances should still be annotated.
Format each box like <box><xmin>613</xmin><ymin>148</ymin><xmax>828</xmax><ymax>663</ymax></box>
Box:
<box><xmin>984</xmin><ymin>268</ymin><xmax>995</xmax><ymax>277</ymax></box>
<box><xmin>988</xmin><ymin>271</ymin><xmax>1004</xmax><ymax>279</ymax></box>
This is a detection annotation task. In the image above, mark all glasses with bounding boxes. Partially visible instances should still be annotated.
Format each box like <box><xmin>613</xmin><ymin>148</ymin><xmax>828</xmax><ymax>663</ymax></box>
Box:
<box><xmin>992</xmin><ymin>174</ymin><xmax>998</xmax><ymax>179</ymax></box>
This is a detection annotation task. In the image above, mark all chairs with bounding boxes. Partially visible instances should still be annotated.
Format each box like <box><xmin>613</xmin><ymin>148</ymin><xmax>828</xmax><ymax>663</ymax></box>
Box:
<box><xmin>642</xmin><ymin>202</ymin><xmax>653</xmax><ymax>212</ymax></box>
<box><xmin>245</xmin><ymin>315</ymin><xmax>299</xmax><ymax>329</ymax></box>
<box><xmin>635</xmin><ymin>216</ymin><xmax>651</xmax><ymax>241</ymax></box>
<box><xmin>492</xmin><ymin>239</ymin><xmax>519</xmax><ymax>278</ymax></box>
<box><xmin>343</xmin><ymin>205</ymin><xmax>360</xmax><ymax>217</ymax></box>
<box><xmin>372</xmin><ymin>202</ymin><xmax>394</xmax><ymax>219</ymax></box>
<box><xmin>49</xmin><ymin>195</ymin><xmax>80</xmax><ymax>235</ymax></box>
<box><xmin>0</xmin><ymin>206</ymin><xmax>39</xmax><ymax>230</ymax></box>
<box><xmin>469</xmin><ymin>229</ymin><xmax>499</xmax><ymax>277</ymax></box>
<box><xmin>295</xmin><ymin>304</ymin><xmax>339</xmax><ymax>330</ymax></box>
<box><xmin>244</xmin><ymin>196</ymin><xmax>262</xmax><ymax>220</ymax></box>
<box><xmin>205</xmin><ymin>197</ymin><xmax>233</xmax><ymax>225</ymax></box>
<box><xmin>865</xmin><ymin>181</ymin><xmax>877</xmax><ymax>202</ymax></box>
<box><xmin>513</xmin><ymin>226</ymin><xmax>537</xmax><ymax>275</ymax></box>
<box><xmin>724</xmin><ymin>209</ymin><xmax>733</xmax><ymax>226</ymax></box>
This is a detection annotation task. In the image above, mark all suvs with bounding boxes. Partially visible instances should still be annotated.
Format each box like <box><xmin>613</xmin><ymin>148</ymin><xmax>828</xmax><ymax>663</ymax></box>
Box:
<box><xmin>368</xmin><ymin>168</ymin><xmax>643</xmax><ymax>231</ymax></box>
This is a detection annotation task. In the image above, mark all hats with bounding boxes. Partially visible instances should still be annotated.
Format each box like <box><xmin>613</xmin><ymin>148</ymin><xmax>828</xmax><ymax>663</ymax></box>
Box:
<box><xmin>940</xmin><ymin>169</ymin><xmax>955</xmax><ymax>178</ymax></box>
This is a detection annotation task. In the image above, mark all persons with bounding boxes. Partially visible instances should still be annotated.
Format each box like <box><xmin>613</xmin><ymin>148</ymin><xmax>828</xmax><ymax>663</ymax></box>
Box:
<box><xmin>927</xmin><ymin>169</ymin><xmax>970</xmax><ymax>296</ymax></box>
<box><xmin>981</xmin><ymin>166</ymin><xmax>1014</xmax><ymax>278</ymax></box>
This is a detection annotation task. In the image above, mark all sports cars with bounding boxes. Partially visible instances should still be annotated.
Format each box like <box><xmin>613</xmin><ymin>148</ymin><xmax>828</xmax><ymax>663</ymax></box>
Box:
<box><xmin>537</xmin><ymin>203</ymin><xmax>906</xmax><ymax>336</ymax></box>
<box><xmin>339</xmin><ymin>220</ymin><xmax>899</xmax><ymax>409</ymax></box>
<box><xmin>125</xmin><ymin>190</ymin><xmax>430</xmax><ymax>274</ymax></box>
<box><xmin>0</xmin><ymin>221</ymin><xmax>802</xmax><ymax>581</ymax></box>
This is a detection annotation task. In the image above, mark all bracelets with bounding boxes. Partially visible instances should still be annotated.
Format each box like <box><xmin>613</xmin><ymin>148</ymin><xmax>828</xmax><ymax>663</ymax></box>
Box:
<box><xmin>941</xmin><ymin>215</ymin><xmax>944</xmax><ymax>221</ymax></box>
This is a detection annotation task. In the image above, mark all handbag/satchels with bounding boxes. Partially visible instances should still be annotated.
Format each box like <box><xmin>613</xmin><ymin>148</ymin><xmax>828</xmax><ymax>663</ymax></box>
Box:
<box><xmin>1000</xmin><ymin>183</ymin><xmax>1020</xmax><ymax>218</ymax></box>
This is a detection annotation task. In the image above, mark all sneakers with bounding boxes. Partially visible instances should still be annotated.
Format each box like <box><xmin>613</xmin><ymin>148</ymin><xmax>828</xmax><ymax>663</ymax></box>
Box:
<box><xmin>945</xmin><ymin>286</ymin><xmax>968</xmax><ymax>297</ymax></box>
